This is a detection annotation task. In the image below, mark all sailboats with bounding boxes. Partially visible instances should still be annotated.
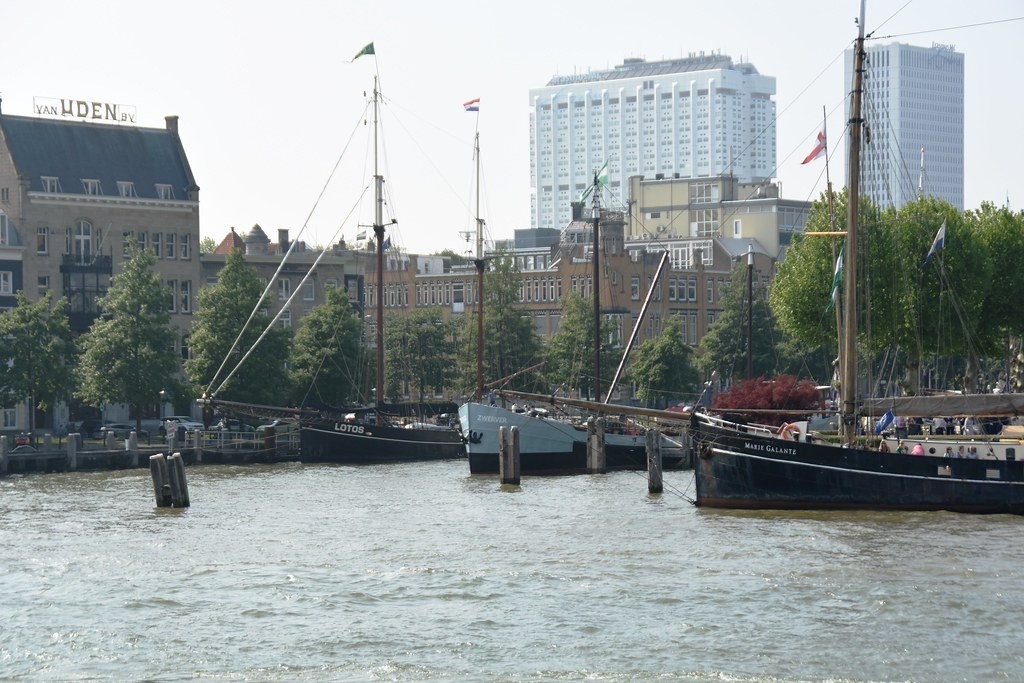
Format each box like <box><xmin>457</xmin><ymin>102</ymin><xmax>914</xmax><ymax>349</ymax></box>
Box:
<box><xmin>490</xmin><ymin>0</ymin><xmax>1024</xmax><ymax>514</ymax></box>
<box><xmin>457</xmin><ymin>169</ymin><xmax>693</xmax><ymax>475</ymax></box>
<box><xmin>196</xmin><ymin>74</ymin><xmax>467</xmax><ymax>465</ymax></box>
<box><xmin>654</xmin><ymin>244</ymin><xmax>840</xmax><ymax>432</ymax></box>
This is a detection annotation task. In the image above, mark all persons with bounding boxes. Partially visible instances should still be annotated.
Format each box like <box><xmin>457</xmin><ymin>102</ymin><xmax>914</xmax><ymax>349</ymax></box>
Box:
<box><xmin>892</xmin><ymin>416</ymin><xmax>974</xmax><ymax>434</ymax></box>
<box><xmin>878</xmin><ymin>439</ymin><xmax>978</xmax><ymax>459</ymax></box>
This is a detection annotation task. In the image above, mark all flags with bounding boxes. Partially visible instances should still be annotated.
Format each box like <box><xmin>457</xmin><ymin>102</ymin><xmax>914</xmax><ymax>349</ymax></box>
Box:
<box><xmin>801</xmin><ymin>118</ymin><xmax>826</xmax><ymax>164</ymax></box>
<box><xmin>923</xmin><ymin>222</ymin><xmax>945</xmax><ymax>266</ymax></box>
<box><xmin>875</xmin><ymin>411</ymin><xmax>894</xmax><ymax>436</ymax></box>
<box><xmin>579</xmin><ymin>157</ymin><xmax>608</xmax><ymax>203</ymax></box>
<box><xmin>357</xmin><ymin>231</ymin><xmax>366</xmax><ymax>239</ymax></box>
<box><xmin>382</xmin><ymin>236</ymin><xmax>390</xmax><ymax>248</ymax></box>
<box><xmin>351</xmin><ymin>42</ymin><xmax>375</xmax><ymax>63</ymax></box>
<box><xmin>463</xmin><ymin>99</ymin><xmax>480</xmax><ymax>111</ymax></box>
<box><xmin>826</xmin><ymin>248</ymin><xmax>843</xmax><ymax>312</ymax></box>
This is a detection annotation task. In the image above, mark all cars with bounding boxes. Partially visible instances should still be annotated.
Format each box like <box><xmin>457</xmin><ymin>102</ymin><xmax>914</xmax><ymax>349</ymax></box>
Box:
<box><xmin>100</xmin><ymin>423</ymin><xmax>149</xmax><ymax>439</ymax></box>
<box><xmin>218</xmin><ymin>420</ymin><xmax>255</xmax><ymax>432</ymax></box>
<box><xmin>256</xmin><ymin>418</ymin><xmax>300</xmax><ymax>433</ymax></box>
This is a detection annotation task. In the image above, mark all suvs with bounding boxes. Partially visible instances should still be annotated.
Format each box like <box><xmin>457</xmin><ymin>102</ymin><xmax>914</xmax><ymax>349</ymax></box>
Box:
<box><xmin>158</xmin><ymin>416</ymin><xmax>205</xmax><ymax>436</ymax></box>
